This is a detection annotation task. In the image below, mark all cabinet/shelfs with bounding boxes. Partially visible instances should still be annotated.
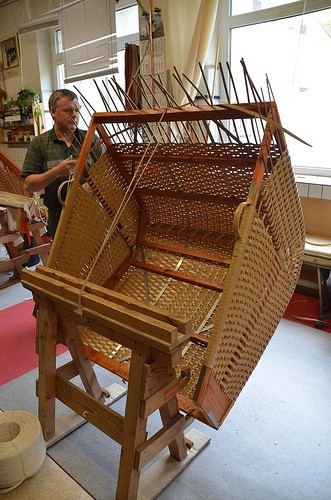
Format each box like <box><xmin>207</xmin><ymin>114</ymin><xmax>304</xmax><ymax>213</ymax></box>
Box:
<box><xmin>0</xmin><ymin>101</ymin><xmax>43</xmax><ymax>143</ymax></box>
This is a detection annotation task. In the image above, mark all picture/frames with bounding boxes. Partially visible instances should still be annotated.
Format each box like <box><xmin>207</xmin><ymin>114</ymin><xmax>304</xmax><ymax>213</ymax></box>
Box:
<box><xmin>0</xmin><ymin>36</ymin><xmax>19</xmax><ymax>70</ymax></box>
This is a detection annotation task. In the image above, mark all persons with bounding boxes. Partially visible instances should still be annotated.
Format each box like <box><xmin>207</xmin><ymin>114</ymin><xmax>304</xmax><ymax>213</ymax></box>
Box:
<box><xmin>20</xmin><ymin>89</ymin><xmax>103</xmax><ymax>240</ymax></box>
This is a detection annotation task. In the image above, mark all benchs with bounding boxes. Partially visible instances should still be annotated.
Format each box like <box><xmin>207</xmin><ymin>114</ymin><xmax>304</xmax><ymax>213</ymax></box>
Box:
<box><xmin>296</xmin><ymin>235</ymin><xmax>331</xmax><ymax>315</ymax></box>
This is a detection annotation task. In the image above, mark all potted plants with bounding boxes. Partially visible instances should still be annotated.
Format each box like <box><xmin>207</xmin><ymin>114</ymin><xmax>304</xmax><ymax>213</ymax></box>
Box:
<box><xmin>4</xmin><ymin>89</ymin><xmax>37</xmax><ymax>119</ymax></box>
